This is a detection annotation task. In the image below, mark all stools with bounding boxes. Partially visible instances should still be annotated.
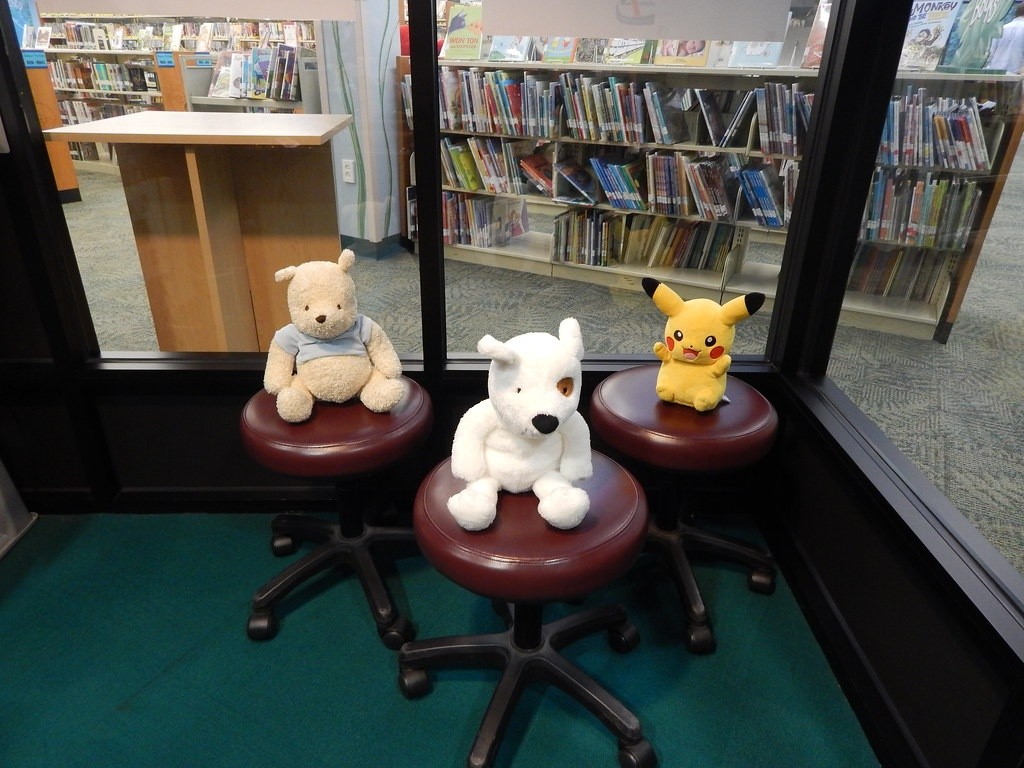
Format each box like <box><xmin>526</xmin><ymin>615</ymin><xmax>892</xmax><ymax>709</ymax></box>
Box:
<box><xmin>589</xmin><ymin>363</ymin><xmax>777</xmax><ymax>654</ymax></box>
<box><xmin>396</xmin><ymin>453</ymin><xmax>661</xmax><ymax>768</ymax></box>
<box><xmin>239</xmin><ymin>376</ymin><xmax>432</xmax><ymax>651</ymax></box>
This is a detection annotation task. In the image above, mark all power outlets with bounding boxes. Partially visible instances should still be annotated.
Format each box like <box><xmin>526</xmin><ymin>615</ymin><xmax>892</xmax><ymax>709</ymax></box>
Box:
<box><xmin>342</xmin><ymin>159</ymin><xmax>355</xmax><ymax>183</ymax></box>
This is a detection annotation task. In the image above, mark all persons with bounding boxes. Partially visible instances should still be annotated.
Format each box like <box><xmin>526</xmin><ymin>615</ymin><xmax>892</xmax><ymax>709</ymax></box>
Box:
<box><xmin>663</xmin><ymin>40</ymin><xmax>705</xmax><ymax>56</ymax></box>
<box><xmin>511</xmin><ymin>210</ymin><xmax>525</xmax><ymax>236</ymax></box>
<box><xmin>985</xmin><ymin>2</ymin><xmax>1024</xmax><ymax>75</ymax></box>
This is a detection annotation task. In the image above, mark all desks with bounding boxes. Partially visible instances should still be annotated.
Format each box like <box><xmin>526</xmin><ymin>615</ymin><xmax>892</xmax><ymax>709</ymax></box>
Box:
<box><xmin>40</xmin><ymin>111</ymin><xmax>353</xmax><ymax>353</ymax></box>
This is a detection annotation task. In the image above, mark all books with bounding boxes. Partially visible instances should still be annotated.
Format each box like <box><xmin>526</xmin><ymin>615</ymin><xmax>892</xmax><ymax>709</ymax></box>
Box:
<box><xmin>45</xmin><ymin>21</ymin><xmax>315</xmax><ymax>165</ymax></box>
<box><xmin>400</xmin><ymin>0</ymin><xmax>832</xmax><ymax>269</ymax></box>
<box><xmin>845</xmin><ymin>0</ymin><xmax>1024</xmax><ymax>303</ymax></box>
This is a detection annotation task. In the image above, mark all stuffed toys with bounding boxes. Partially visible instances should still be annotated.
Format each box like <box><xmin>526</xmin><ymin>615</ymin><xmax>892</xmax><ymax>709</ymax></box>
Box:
<box><xmin>263</xmin><ymin>248</ymin><xmax>404</xmax><ymax>422</ymax></box>
<box><xmin>446</xmin><ymin>317</ymin><xmax>594</xmax><ymax>531</ymax></box>
<box><xmin>642</xmin><ymin>277</ymin><xmax>765</xmax><ymax>411</ymax></box>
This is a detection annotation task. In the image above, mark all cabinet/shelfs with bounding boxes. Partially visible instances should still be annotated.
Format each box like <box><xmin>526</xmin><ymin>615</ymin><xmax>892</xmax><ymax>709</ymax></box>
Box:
<box><xmin>394</xmin><ymin>53</ymin><xmax>1024</xmax><ymax>346</ymax></box>
<box><xmin>41</xmin><ymin>12</ymin><xmax>320</xmax><ymax>177</ymax></box>
